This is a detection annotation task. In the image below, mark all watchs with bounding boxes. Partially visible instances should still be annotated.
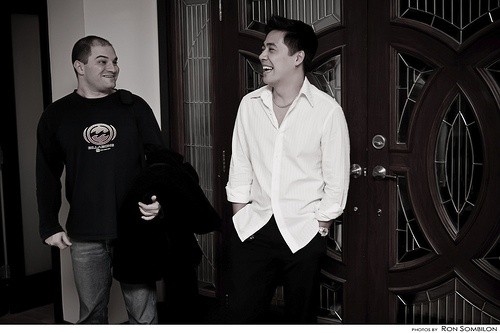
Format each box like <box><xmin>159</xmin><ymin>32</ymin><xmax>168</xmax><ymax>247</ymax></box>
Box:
<box><xmin>318</xmin><ymin>226</ymin><xmax>328</xmax><ymax>236</ymax></box>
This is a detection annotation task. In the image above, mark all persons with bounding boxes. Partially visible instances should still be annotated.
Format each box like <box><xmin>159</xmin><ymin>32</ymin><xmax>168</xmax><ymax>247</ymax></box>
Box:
<box><xmin>35</xmin><ymin>35</ymin><xmax>171</xmax><ymax>325</ymax></box>
<box><xmin>225</xmin><ymin>15</ymin><xmax>351</xmax><ymax>324</ymax></box>
